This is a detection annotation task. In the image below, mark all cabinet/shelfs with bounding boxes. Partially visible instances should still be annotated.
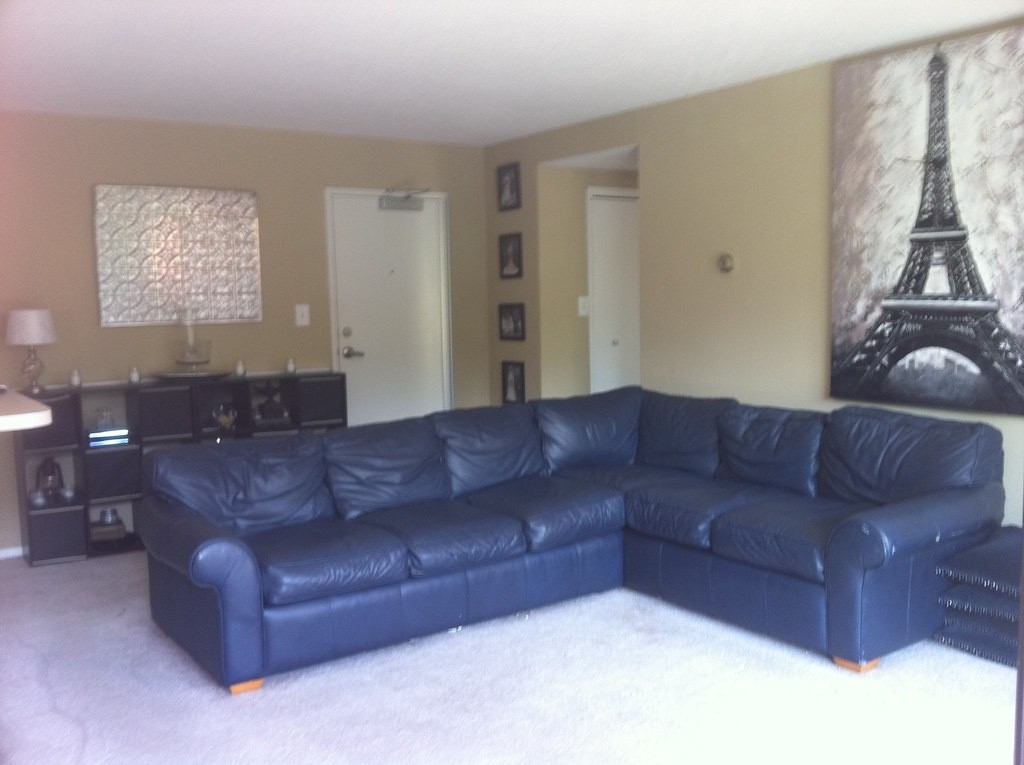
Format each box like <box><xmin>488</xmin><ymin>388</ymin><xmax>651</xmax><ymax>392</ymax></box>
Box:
<box><xmin>17</xmin><ymin>368</ymin><xmax>344</xmax><ymax>568</ymax></box>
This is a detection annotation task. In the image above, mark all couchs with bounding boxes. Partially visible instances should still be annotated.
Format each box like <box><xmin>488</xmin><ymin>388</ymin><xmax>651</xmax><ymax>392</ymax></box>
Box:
<box><xmin>133</xmin><ymin>463</ymin><xmax>1005</xmax><ymax>694</ymax></box>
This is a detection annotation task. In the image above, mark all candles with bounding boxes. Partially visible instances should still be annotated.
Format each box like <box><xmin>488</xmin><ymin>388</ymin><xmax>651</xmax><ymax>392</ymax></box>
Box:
<box><xmin>129</xmin><ymin>368</ymin><xmax>138</xmax><ymax>383</ymax></box>
<box><xmin>235</xmin><ymin>361</ymin><xmax>244</xmax><ymax>375</ymax></box>
<box><xmin>286</xmin><ymin>358</ymin><xmax>294</xmax><ymax>371</ymax></box>
<box><xmin>69</xmin><ymin>370</ymin><xmax>79</xmax><ymax>386</ymax></box>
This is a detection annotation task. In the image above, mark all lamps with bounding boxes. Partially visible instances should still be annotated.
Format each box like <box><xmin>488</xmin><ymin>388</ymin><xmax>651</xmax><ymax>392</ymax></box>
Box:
<box><xmin>4</xmin><ymin>307</ymin><xmax>57</xmax><ymax>399</ymax></box>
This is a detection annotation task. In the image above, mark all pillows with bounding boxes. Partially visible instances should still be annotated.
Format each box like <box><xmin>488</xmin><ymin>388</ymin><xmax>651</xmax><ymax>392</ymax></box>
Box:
<box><xmin>717</xmin><ymin>406</ymin><xmax>826</xmax><ymax>498</ymax></box>
<box><xmin>323</xmin><ymin>418</ymin><xmax>452</xmax><ymax>521</ymax></box>
<box><xmin>140</xmin><ymin>435</ymin><xmax>338</xmax><ymax>539</ymax></box>
<box><xmin>425</xmin><ymin>405</ymin><xmax>549</xmax><ymax>496</ymax></box>
<box><xmin>527</xmin><ymin>382</ymin><xmax>642</xmax><ymax>478</ymax></box>
<box><xmin>639</xmin><ymin>388</ymin><xmax>741</xmax><ymax>477</ymax></box>
<box><xmin>815</xmin><ymin>403</ymin><xmax>1001</xmax><ymax>506</ymax></box>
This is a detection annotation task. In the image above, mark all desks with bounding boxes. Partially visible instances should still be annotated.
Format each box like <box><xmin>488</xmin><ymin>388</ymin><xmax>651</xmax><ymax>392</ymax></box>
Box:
<box><xmin>0</xmin><ymin>388</ymin><xmax>55</xmax><ymax>435</ymax></box>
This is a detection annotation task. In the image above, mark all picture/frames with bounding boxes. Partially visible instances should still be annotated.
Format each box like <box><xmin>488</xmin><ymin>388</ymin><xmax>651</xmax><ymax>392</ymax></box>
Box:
<box><xmin>498</xmin><ymin>230</ymin><xmax>522</xmax><ymax>279</ymax></box>
<box><xmin>498</xmin><ymin>301</ymin><xmax>524</xmax><ymax>342</ymax></box>
<box><xmin>501</xmin><ymin>360</ymin><xmax>526</xmax><ymax>406</ymax></box>
<box><xmin>495</xmin><ymin>162</ymin><xmax>520</xmax><ymax>213</ymax></box>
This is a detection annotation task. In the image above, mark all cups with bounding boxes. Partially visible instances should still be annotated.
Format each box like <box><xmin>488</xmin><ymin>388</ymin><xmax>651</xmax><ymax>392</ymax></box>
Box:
<box><xmin>29</xmin><ymin>489</ymin><xmax>47</xmax><ymax>508</ymax></box>
<box><xmin>100</xmin><ymin>509</ymin><xmax>118</xmax><ymax>525</ymax></box>
<box><xmin>128</xmin><ymin>366</ymin><xmax>141</xmax><ymax>384</ymax></box>
<box><xmin>68</xmin><ymin>367</ymin><xmax>82</xmax><ymax>388</ymax></box>
<box><xmin>234</xmin><ymin>359</ymin><xmax>246</xmax><ymax>376</ymax></box>
<box><xmin>95</xmin><ymin>407</ymin><xmax>113</xmax><ymax>423</ymax></box>
<box><xmin>285</xmin><ymin>358</ymin><xmax>296</xmax><ymax>375</ymax></box>
<box><xmin>61</xmin><ymin>479</ymin><xmax>80</xmax><ymax>505</ymax></box>
